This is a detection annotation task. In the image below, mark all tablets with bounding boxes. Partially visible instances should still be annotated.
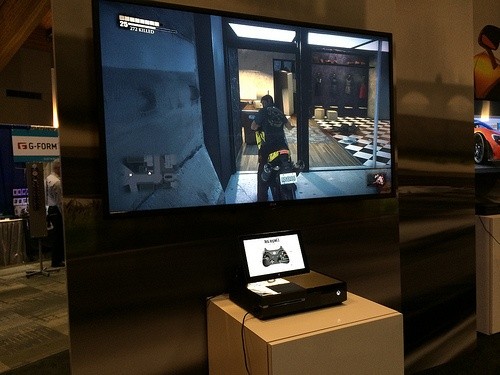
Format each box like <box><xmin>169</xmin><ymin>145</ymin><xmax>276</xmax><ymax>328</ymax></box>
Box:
<box><xmin>238</xmin><ymin>228</ymin><xmax>310</xmax><ymax>282</ymax></box>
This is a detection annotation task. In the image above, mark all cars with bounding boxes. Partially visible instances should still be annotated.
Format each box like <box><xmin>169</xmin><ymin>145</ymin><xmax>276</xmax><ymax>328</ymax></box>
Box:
<box><xmin>473</xmin><ymin>118</ymin><xmax>500</xmax><ymax>165</ymax></box>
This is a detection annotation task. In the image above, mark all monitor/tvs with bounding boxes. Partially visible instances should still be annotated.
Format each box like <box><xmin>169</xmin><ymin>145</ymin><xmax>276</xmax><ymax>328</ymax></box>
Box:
<box><xmin>92</xmin><ymin>0</ymin><xmax>397</xmax><ymax>221</ymax></box>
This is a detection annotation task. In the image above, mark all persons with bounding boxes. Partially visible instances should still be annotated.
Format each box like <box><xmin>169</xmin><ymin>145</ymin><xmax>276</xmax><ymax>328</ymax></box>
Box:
<box><xmin>250</xmin><ymin>94</ymin><xmax>306</xmax><ymax>203</ymax></box>
<box><xmin>46</xmin><ymin>159</ymin><xmax>66</xmax><ymax>267</ymax></box>
